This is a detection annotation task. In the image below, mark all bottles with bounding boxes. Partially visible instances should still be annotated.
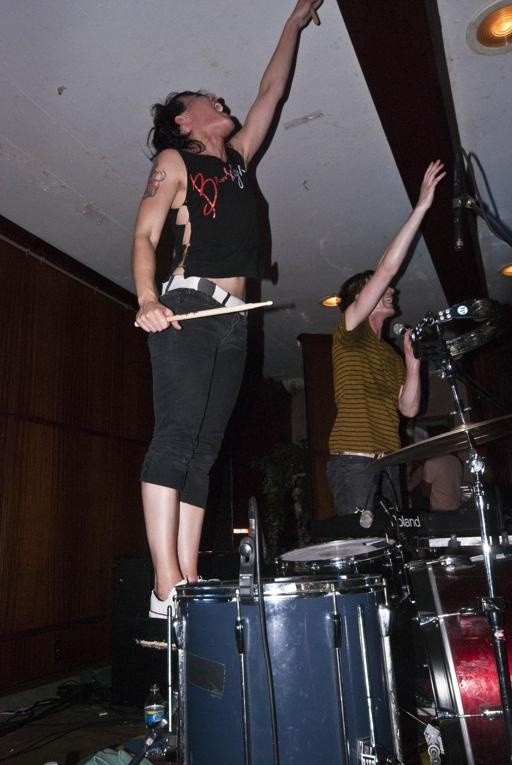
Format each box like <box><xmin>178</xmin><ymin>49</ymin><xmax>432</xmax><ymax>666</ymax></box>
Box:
<box><xmin>447</xmin><ymin>535</ymin><xmax>461</xmax><ymax>550</ymax></box>
<box><xmin>142</xmin><ymin>682</ymin><xmax>178</xmax><ymax>760</ymax></box>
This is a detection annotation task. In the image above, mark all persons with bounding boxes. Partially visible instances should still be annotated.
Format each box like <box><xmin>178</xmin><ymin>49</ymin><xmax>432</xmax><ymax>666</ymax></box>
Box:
<box><xmin>420</xmin><ymin>450</ymin><xmax>461</xmax><ymax>514</ymax></box>
<box><xmin>327</xmin><ymin>158</ymin><xmax>449</xmax><ymax>519</ymax></box>
<box><xmin>130</xmin><ymin>0</ymin><xmax>329</xmax><ymax>621</ymax></box>
<box><xmin>311</xmin><ymin>429</ymin><xmax>338</xmax><ymax>522</ymax></box>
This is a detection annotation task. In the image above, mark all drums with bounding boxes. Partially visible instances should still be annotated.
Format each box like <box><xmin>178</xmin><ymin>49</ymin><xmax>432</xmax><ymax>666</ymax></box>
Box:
<box><xmin>173</xmin><ymin>574</ymin><xmax>405</xmax><ymax>764</ymax></box>
<box><xmin>414</xmin><ymin>609</ymin><xmax>511</xmax><ymax>764</ymax></box>
<box><xmin>274</xmin><ymin>535</ymin><xmax>410</xmax><ymax>621</ymax></box>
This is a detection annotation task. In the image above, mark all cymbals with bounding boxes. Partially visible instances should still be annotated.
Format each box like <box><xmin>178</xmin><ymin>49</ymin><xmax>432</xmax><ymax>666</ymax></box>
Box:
<box><xmin>376</xmin><ymin>415</ymin><xmax>512</xmax><ymax>465</ymax></box>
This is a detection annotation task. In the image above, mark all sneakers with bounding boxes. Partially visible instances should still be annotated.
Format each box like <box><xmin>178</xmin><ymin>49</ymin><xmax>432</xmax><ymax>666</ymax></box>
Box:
<box><xmin>148</xmin><ymin>575</ymin><xmax>220</xmax><ymax>620</ymax></box>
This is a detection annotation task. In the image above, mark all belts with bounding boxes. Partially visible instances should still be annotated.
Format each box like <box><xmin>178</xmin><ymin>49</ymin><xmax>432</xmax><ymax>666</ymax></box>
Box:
<box><xmin>330</xmin><ymin>450</ymin><xmax>392</xmax><ymax>459</ymax></box>
<box><xmin>162</xmin><ymin>275</ymin><xmax>248</xmax><ymax>317</ymax></box>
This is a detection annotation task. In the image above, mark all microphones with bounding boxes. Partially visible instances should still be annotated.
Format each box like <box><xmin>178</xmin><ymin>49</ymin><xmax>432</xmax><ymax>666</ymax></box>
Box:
<box><xmin>359</xmin><ymin>472</ymin><xmax>383</xmax><ymax>529</ymax></box>
<box><xmin>393</xmin><ymin>322</ymin><xmax>406</xmax><ymax>336</ymax></box>
